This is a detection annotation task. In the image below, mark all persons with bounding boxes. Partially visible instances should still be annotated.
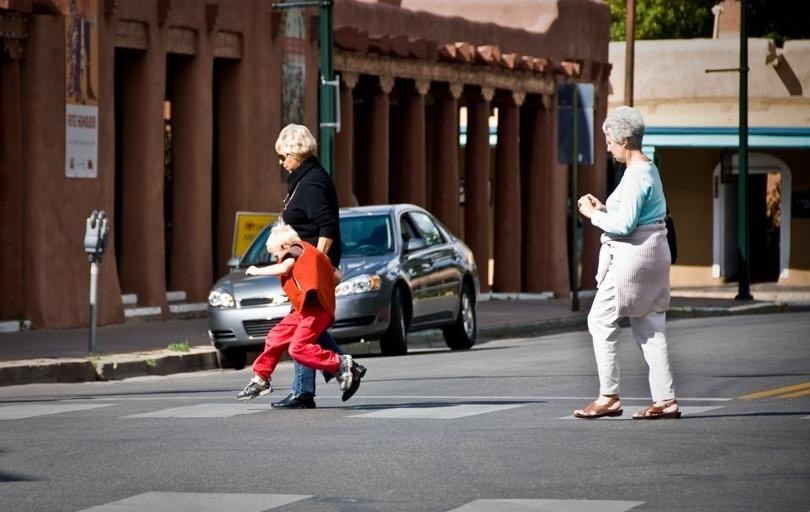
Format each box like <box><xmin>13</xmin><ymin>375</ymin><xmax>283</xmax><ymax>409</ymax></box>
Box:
<box><xmin>234</xmin><ymin>220</ymin><xmax>354</xmax><ymax>401</ymax></box>
<box><xmin>271</xmin><ymin>122</ymin><xmax>367</xmax><ymax>411</ymax></box>
<box><xmin>573</xmin><ymin>105</ymin><xmax>682</xmax><ymax>421</ymax></box>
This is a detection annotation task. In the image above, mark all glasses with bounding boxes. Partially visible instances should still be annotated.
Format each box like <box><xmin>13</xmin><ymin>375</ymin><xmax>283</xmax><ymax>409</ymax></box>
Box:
<box><xmin>279</xmin><ymin>155</ymin><xmax>288</xmax><ymax>161</ymax></box>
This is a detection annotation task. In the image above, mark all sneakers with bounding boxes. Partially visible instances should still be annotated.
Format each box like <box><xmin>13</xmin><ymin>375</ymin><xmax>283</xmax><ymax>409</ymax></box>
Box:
<box><xmin>236</xmin><ymin>379</ymin><xmax>271</xmax><ymax>402</ymax></box>
<box><xmin>338</xmin><ymin>354</ymin><xmax>353</xmax><ymax>390</ymax></box>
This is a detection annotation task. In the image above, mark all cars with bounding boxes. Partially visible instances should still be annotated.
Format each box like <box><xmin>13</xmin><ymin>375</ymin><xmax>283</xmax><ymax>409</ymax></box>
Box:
<box><xmin>206</xmin><ymin>202</ymin><xmax>481</xmax><ymax>371</ymax></box>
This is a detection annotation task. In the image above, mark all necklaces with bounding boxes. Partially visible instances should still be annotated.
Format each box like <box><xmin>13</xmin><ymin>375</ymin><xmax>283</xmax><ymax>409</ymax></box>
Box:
<box><xmin>281</xmin><ymin>183</ymin><xmax>299</xmax><ymax>211</ymax></box>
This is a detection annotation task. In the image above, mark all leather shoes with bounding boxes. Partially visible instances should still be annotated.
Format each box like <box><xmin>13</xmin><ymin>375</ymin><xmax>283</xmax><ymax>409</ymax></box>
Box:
<box><xmin>341</xmin><ymin>362</ymin><xmax>367</xmax><ymax>401</ymax></box>
<box><xmin>271</xmin><ymin>393</ymin><xmax>316</xmax><ymax>409</ymax></box>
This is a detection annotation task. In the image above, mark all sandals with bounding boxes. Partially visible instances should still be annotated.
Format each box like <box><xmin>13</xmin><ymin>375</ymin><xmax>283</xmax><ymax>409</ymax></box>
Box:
<box><xmin>633</xmin><ymin>401</ymin><xmax>682</xmax><ymax>418</ymax></box>
<box><xmin>574</xmin><ymin>396</ymin><xmax>623</xmax><ymax>419</ymax></box>
